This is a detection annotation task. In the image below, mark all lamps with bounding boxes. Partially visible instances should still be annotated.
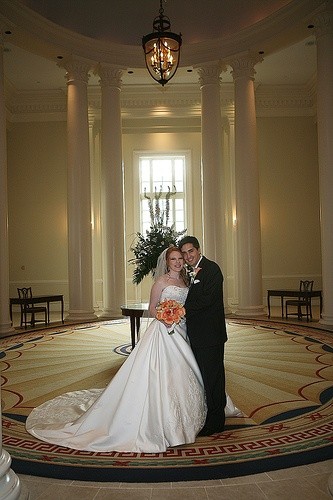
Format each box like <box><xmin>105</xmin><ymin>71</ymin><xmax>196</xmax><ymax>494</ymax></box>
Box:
<box><xmin>142</xmin><ymin>0</ymin><xmax>182</xmax><ymax>87</ymax></box>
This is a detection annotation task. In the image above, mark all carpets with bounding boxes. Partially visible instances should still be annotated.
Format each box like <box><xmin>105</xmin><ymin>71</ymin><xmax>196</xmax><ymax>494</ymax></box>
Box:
<box><xmin>0</xmin><ymin>317</ymin><xmax>333</xmax><ymax>483</ymax></box>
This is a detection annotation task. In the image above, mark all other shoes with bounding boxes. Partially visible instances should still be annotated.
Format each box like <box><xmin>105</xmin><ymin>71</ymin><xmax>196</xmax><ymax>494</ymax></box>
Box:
<box><xmin>197</xmin><ymin>423</ymin><xmax>225</xmax><ymax>436</ymax></box>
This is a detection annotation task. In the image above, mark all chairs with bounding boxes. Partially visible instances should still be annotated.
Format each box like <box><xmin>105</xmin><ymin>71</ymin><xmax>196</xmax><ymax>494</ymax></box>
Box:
<box><xmin>286</xmin><ymin>280</ymin><xmax>314</xmax><ymax>320</ymax></box>
<box><xmin>17</xmin><ymin>287</ymin><xmax>47</xmax><ymax>330</ymax></box>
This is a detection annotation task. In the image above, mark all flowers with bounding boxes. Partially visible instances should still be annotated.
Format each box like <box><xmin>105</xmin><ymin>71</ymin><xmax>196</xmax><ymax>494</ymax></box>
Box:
<box><xmin>188</xmin><ymin>267</ymin><xmax>201</xmax><ymax>285</ymax></box>
<box><xmin>127</xmin><ymin>185</ymin><xmax>188</xmax><ymax>286</ymax></box>
<box><xmin>155</xmin><ymin>299</ymin><xmax>186</xmax><ymax>335</ymax></box>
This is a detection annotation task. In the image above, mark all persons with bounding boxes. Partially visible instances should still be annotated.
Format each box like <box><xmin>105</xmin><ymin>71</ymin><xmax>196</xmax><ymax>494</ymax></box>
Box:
<box><xmin>178</xmin><ymin>236</ymin><xmax>228</xmax><ymax>437</ymax></box>
<box><xmin>148</xmin><ymin>246</ymin><xmax>192</xmax><ymax>446</ymax></box>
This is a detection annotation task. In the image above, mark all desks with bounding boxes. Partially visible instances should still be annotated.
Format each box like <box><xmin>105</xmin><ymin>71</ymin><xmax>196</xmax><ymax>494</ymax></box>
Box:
<box><xmin>267</xmin><ymin>289</ymin><xmax>323</xmax><ymax>319</ymax></box>
<box><xmin>120</xmin><ymin>303</ymin><xmax>150</xmax><ymax>351</ymax></box>
<box><xmin>10</xmin><ymin>294</ymin><xmax>65</xmax><ymax>327</ymax></box>
